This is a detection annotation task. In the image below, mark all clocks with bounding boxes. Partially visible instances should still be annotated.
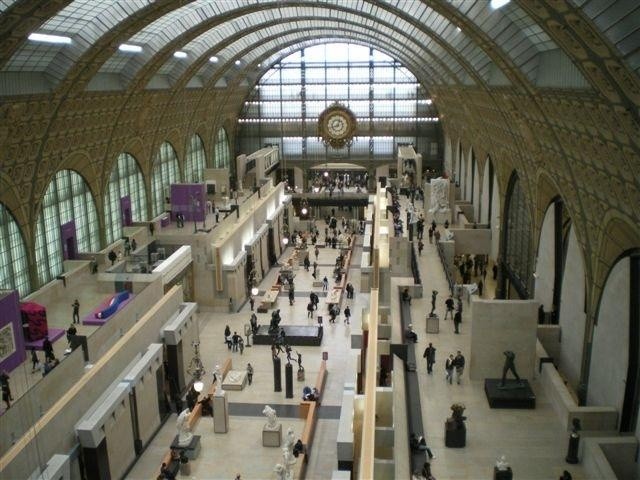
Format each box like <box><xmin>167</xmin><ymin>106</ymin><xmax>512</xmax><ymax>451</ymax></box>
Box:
<box><xmin>314</xmin><ymin>100</ymin><xmax>359</xmax><ymax>150</ymax></box>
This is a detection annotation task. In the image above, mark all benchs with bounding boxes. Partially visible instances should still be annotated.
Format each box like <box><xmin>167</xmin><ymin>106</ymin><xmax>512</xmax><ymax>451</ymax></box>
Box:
<box><xmin>153</xmin><ymin>358</ymin><xmax>233</xmax><ymax>479</ymax></box>
<box><xmin>399</xmin><ymin>290</ymin><xmax>429</xmax><ymax>479</ymax></box>
<box><xmin>260</xmin><ymin>227</ymin><xmax>355</xmax><ymax>314</ymax></box>
<box><xmin>286</xmin><ymin>356</ymin><xmax>328</xmax><ymax>480</ymax></box>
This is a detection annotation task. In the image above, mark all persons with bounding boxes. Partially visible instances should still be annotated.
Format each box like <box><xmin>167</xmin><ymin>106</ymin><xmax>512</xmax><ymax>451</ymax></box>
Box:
<box><xmin>503</xmin><ymin>350</ymin><xmax>523</xmax><ymax>387</ymax></box>
<box><xmin>155</xmin><ymin>159</ymin><xmax>499</xmax><ymax>480</ymax></box>
<box><xmin>30</xmin><ymin>347</ymin><xmax>41</xmax><ymax>371</ymax></box>
<box><xmin>131</xmin><ymin>239</ymin><xmax>139</xmax><ymax>253</ymax></box>
<box><xmin>538</xmin><ymin>303</ymin><xmax>546</xmax><ymax>323</ymax></box>
<box><xmin>89</xmin><ymin>256</ymin><xmax>98</xmax><ymax>274</ymax></box>
<box><xmin>149</xmin><ymin>221</ymin><xmax>154</xmax><ymax>234</ymax></box>
<box><xmin>107</xmin><ymin>249</ymin><xmax>117</xmax><ymax>268</ymax></box>
<box><xmin>117</xmin><ymin>252</ymin><xmax>123</xmax><ymax>262</ymax></box>
<box><xmin>66</xmin><ymin>324</ymin><xmax>76</xmax><ymax>345</ymax></box>
<box><xmin>41</xmin><ymin>335</ymin><xmax>55</xmax><ymax>365</ymax></box>
<box><xmin>123</xmin><ymin>240</ymin><xmax>130</xmax><ymax>257</ymax></box>
<box><xmin>71</xmin><ymin>299</ymin><xmax>80</xmax><ymax>324</ymax></box>
<box><xmin>0</xmin><ymin>383</ymin><xmax>12</xmax><ymax>410</ymax></box>
<box><xmin>1</xmin><ymin>370</ymin><xmax>14</xmax><ymax>401</ymax></box>
